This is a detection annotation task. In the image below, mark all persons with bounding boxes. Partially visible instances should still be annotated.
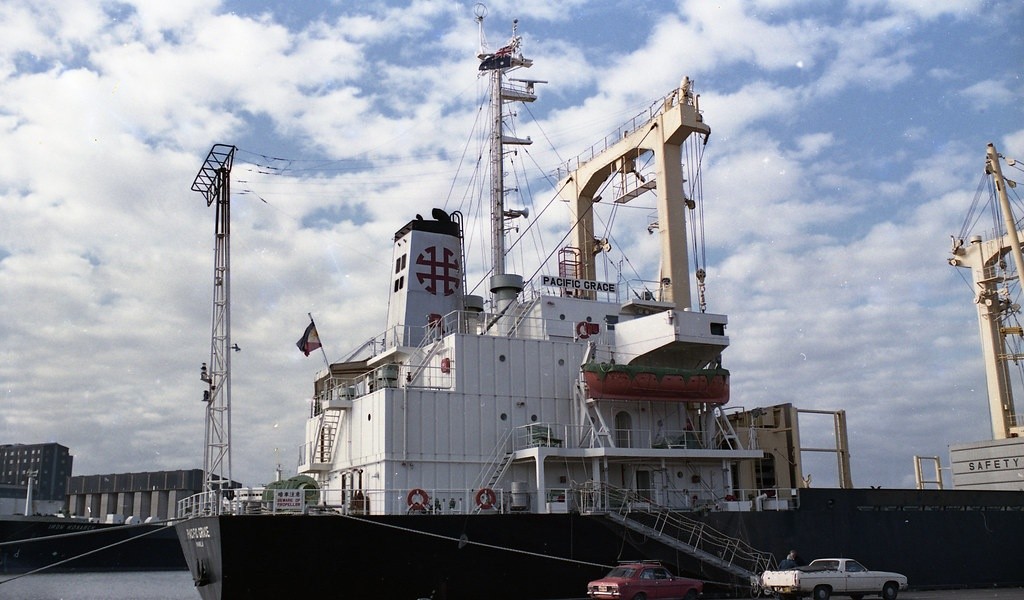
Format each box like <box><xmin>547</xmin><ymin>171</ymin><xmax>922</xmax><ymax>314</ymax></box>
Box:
<box><xmin>779</xmin><ymin>550</ymin><xmax>799</xmax><ymax>571</ymax></box>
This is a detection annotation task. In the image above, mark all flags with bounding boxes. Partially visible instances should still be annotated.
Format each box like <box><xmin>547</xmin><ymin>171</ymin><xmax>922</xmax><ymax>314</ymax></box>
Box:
<box><xmin>295</xmin><ymin>321</ymin><xmax>322</xmax><ymax>360</ymax></box>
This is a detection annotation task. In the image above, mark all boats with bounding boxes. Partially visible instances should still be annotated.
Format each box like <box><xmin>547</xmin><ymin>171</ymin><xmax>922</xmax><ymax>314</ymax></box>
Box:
<box><xmin>175</xmin><ymin>2</ymin><xmax>1024</xmax><ymax>600</ymax></box>
<box><xmin>0</xmin><ymin>468</ymin><xmax>188</xmax><ymax>574</ymax></box>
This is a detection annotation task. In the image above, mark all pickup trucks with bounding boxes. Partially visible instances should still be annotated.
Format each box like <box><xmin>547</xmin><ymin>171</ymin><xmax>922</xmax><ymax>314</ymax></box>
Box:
<box><xmin>761</xmin><ymin>559</ymin><xmax>908</xmax><ymax>600</ymax></box>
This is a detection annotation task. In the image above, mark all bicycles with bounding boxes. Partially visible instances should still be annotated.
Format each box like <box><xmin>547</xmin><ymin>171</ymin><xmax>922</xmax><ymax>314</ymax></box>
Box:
<box><xmin>750</xmin><ymin>572</ymin><xmax>781</xmax><ymax>600</ymax></box>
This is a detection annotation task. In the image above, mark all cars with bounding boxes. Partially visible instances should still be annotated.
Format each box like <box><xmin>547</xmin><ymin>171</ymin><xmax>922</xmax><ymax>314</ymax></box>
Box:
<box><xmin>587</xmin><ymin>560</ymin><xmax>705</xmax><ymax>600</ymax></box>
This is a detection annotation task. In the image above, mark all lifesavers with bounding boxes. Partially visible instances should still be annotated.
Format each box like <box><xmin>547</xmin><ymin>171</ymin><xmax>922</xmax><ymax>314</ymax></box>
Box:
<box><xmin>476</xmin><ymin>488</ymin><xmax>497</xmax><ymax>509</ymax></box>
<box><xmin>576</xmin><ymin>321</ymin><xmax>592</xmax><ymax>339</ymax></box>
<box><xmin>407</xmin><ymin>488</ymin><xmax>429</xmax><ymax>511</ymax></box>
